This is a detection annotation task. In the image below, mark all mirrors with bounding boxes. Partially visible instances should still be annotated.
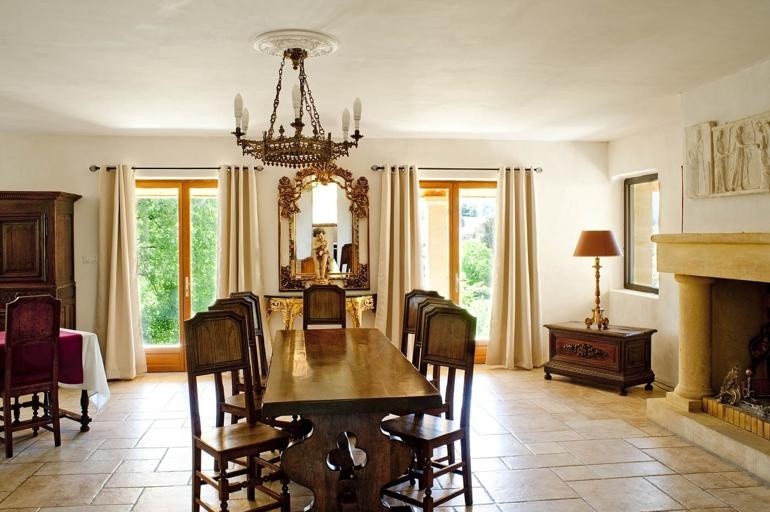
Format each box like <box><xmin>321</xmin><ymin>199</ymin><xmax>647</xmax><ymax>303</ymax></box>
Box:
<box><xmin>278</xmin><ymin>165</ymin><xmax>371</xmax><ymax>294</ymax></box>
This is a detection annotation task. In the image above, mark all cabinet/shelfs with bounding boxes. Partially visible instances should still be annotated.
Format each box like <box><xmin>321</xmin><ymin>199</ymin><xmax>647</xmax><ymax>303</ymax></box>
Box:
<box><xmin>0</xmin><ymin>190</ymin><xmax>83</xmax><ymax>330</ymax></box>
<box><xmin>541</xmin><ymin>321</ymin><xmax>658</xmax><ymax>396</ymax></box>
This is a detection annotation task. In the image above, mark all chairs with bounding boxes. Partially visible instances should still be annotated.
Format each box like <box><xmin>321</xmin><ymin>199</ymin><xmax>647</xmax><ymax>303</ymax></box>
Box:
<box><xmin>2</xmin><ymin>293</ymin><xmax>62</xmax><ymax>459</ymax></box>
<box><xmin>303</xmin><ymin>285</ymin><xmax>348</xmax><ymax>329</ymax></box>
<box><xmin>379</xmin><ymin>291</ymin><xmax>476</xmax><ymax>512</ymax></box>
<box><xmin>301</xmin><ymin>259</ymin><xmax>328</xmax><ymax>275</ymax></box>
<box><xmin>339</xmin><ymin>243</ymin><xmax>354</xmax><ymax>272</ymax></box>
<box><xmin>184</xmin><ymin>291</ymin><xmax>306</xmax><ymax>511</ymax></box>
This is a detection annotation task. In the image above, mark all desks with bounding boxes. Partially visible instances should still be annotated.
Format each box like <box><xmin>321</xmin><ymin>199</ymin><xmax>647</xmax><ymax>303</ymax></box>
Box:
<box><xmin>261</xmin><ymin>327</ymin><xmax>443</xmax><ymax>512</ymax></box>
<box><xmin>0</xmin><ymin>328</ymin><xmax>97</xmax><ymax>438</ymax></box>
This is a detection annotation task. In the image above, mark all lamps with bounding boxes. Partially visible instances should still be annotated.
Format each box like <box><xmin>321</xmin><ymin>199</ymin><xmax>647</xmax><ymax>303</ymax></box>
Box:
<box><xmin>573</xmin><ymin>229</ymin><xmax>624</xmax><ymax>331</ymax></box>
<box><xmin>230</xmin><ymin>28</ymin><xmax>364</xmax><ymax>169</ymax></box>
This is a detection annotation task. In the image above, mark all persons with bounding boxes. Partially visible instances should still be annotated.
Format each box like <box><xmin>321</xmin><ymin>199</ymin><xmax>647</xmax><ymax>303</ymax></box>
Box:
<box><xmin>714</xmin><ymin>131</ymin><xmax>728</xmax><ymax>193</ymax></box>
<box><xmin>730</xmin><ymin>127</ymin><xmax>749</xmax><ymax>191</ymax></box>
<box><xmin>753</xmin><ymin>120</ymin><xmax>770</xmax><ymax>189</ymax></box>
<box><xmin>312</xmin><ymin>229</ymin><xmax>330</xmax><ymax>279</ymax></box>
